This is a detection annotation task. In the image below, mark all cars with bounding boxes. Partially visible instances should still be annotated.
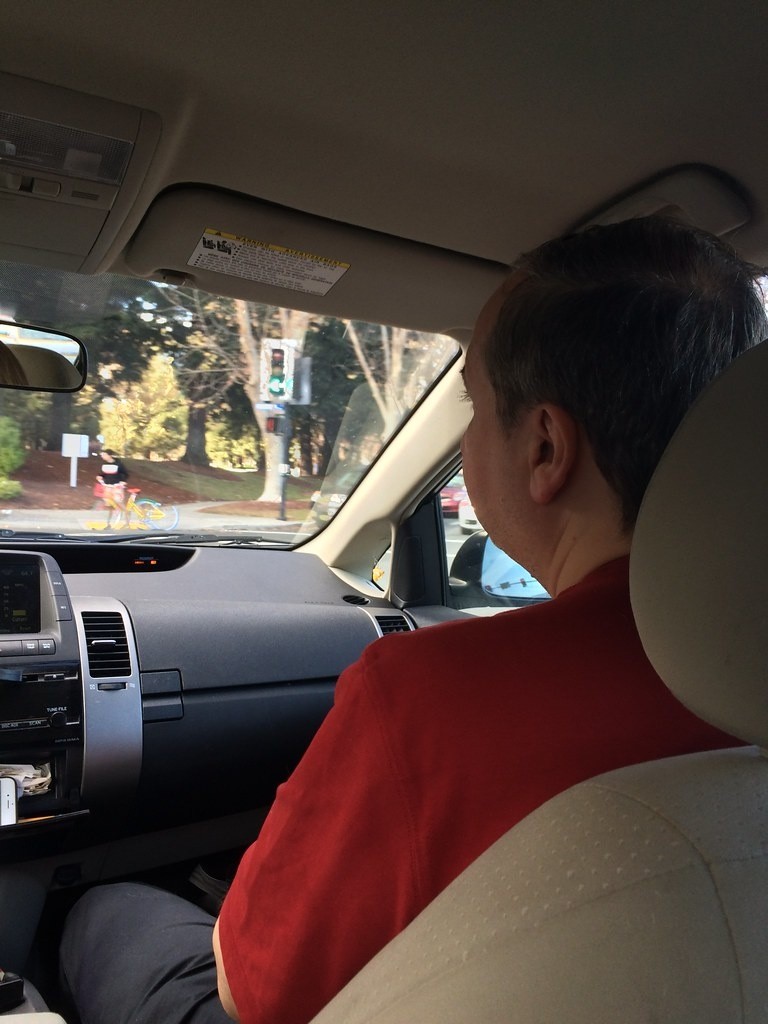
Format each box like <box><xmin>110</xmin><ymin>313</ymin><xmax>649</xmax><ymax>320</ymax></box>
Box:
<box><xmin>458</xmin><ymin>497</ymin><xmax>488</xmax><ymax>535</ymax></box>
<box><xmin>439</xmin><ymin>472</ymin><xmax>465</xmax><ymax>517</ymax></box>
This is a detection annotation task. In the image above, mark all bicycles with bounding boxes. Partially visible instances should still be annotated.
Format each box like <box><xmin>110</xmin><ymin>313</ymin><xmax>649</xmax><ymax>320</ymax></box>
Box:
<box><xmin>87</xmin><ymin>474</ymin><xmax>164</xmax><ymax>529</ymax></box>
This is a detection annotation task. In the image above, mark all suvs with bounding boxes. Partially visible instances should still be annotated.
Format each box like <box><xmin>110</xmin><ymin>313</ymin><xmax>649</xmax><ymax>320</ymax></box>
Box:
<box><xmin>309</xmin><ymin>463</ymin><xmax>370</xmax><ymax>530</ymax></box>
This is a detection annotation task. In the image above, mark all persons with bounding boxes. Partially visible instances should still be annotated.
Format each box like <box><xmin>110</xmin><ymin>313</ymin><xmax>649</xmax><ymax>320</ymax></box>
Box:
<box><xmin>95</xmin><ymin>450</ymin><xmax>127</xmax><ymax>508</ymax></box>
<box><xmin>59</xmin><ymin>201</ymin><xmax>767</xmax><ymax>1024</ymax></box>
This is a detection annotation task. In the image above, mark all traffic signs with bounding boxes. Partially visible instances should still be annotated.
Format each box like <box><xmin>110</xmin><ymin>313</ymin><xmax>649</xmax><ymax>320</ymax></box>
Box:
<box><xmin>270</xmin><ymin>349</ymin><xmax>285</xmax><ymax>397</ymax></box>
<box><xmin>264</xmin><ymin>416</ymin><xmax>292</xmax><ymax>436</ymax></box>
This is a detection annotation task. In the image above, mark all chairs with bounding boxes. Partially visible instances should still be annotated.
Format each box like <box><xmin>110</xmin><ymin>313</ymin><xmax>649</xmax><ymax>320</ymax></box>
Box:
<box><xmin>315</xmin><ymin>333</ymin><xmax>767</xmax><ymax>1024</ymax></box>
